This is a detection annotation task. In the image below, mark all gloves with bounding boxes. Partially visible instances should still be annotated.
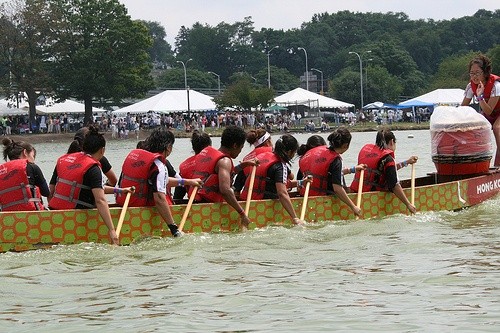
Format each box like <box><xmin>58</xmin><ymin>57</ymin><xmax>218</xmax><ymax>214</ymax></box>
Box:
<box><xmin>168</xmin><ymin>223</ymin><xmax>184</xmax><ymax>238</ymax></box>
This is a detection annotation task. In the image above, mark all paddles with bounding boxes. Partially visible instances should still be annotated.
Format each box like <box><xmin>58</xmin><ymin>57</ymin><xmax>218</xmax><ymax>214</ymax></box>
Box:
<box><xmin>355</xmin><ymin>164</ymin><xmax>367</xmax><ymax>222</ymax></box>
<box><xmin>178</xmin><ymin>185</ymin><xmax>199</xmax><ymax>231</ymax></box>
<box><xmin>411</xmin><ymin>157</ymin><xmax>418</xmax><ymax>216</ymax></box>
<box><xmin>300</xmin><ymin>175</ymin><xmax>313</xmax><ymax>222</ymax></box>
<box><xmin>242</xmin><ymin>165</ymin><xmax>256</xmax><ymax>232</ymax></box>
<box><xmin>114</xmin><ymin>187</ymin><xmax>135</xmax><ymax>242</ymax></box>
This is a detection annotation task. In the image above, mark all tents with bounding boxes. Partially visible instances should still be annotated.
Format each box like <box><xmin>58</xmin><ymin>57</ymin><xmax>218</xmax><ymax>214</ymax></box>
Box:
<box><xmin>399</xmin><ymin>89</ymin><xmax>479</xmax><ymax>106</ymax></box>
<box><xmin>266</xmin><ymin>104</ymin><xmax>289</xmax><ymax>121</ymax></box>
<box><xmin>112</xmin><ymin>89</ymin><xmax>271</xmax><ymax>117</ymax></box>
<box><xmin>245</xmin><ymin>87</ymin><xmax>355</xmax><ymax>114</ymax></box>
<box><xmin>0</xmin><ymin>91</ymin><xmax>107</xmax><ymax>115</ymax></box>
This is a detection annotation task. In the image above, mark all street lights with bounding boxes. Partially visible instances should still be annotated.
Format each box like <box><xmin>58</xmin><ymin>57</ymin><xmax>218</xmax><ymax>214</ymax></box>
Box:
<box><xmin>310</xmin><ymin>68</ymin><xmax>323</xmax><ymax>92</ymax></box>
<box><xmin>176</xmin><ymin>59</ymin><xmax>192</xmax><ymax>90</ymax></box>
<box><xmin>365</xmin><ymin>58</ymin><xmax>373</xmax><ymax>105</ymax></box>
<box><xmin>298</xmin><ymin>47</ymin><xmax>308</xmax><ymax>91</ymax></box>
<box><xmin>208</xmin><ymin>72</ymin><xmax>221</xmax><ymax>97</ymax></box>
<box><xmin>348</xmin><ymin>51</ymin><xmax>372</xmax><ymax>108</ymax></box>
<box><xmin>267</xmin><ymin>46</ymin><xmax>280</xmax><ymax>89</ymax></box>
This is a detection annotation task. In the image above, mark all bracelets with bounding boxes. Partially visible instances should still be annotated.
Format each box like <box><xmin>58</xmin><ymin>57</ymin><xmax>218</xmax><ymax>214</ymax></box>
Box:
<box><xmin>297</xmin><ymin>180</ymin><xmax>303</xmax><ymax>187</ymax></box>
<box><xmin>177</xmin><ymin>178</ymin><xmax>184</xmax><ymax>186</ymax></box>
<box><xmin>399</xmin><ymin>159</ymin><xmax>409</xmax><ymax>168</ymax></box>
<box><xmin>478</xmin><ymin>95</ymin><xmax>484</xmax><ymax>101</ymax></box>
<box><xmin>239</xmin><ymin>209</ymin><xmax>245</xmax><ymax>215</ymax></box>
<box><xmin>115</xmin><ymin>188</ymin><xmax>122</xmax><ymax>193</ymax></box>
<box><xmin>348</xmin><ymin>166</ymin><xmax>356</xmax><ymax>173</ymax></box>
<box><xmin>235</xmin><ymin>164</ymin><xmax>242</xmax><ymax>173</ymax></box>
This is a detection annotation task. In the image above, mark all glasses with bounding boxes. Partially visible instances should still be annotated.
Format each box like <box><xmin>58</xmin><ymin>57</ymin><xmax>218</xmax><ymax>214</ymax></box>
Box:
<box><xmin>470</xmin><ymin>71</ymin><xmax>483</xmax><ymax>76</ymax></box>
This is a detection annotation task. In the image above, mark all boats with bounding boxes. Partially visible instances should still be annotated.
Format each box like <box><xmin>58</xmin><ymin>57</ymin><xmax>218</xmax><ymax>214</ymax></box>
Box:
<box><xmin>0</xmin><ymin>167</ymin><xmax>500</xmax><ymax>254</ymax></box>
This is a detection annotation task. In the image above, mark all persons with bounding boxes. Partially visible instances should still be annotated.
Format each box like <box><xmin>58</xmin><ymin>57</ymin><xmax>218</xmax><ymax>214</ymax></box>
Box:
<box><xmin>136</xmin><ymin>142</ymin><xmax>181</xmax><ymax>179</ymax></box>
<box><xmin>50</xmin><ymin>126</ymin><xmax>134</xmax><ymax>244</ymax></box>
<box><xmin>0</xmin><ymin>106</ymin><xmax>430</xmax><ymax>136</ymax></box>
<box><xmin>173</xmin><ymin>128</ymin><xmax>418</xmax><ymax>225</ymax></box>
<box><xmin>0</xmin><ymin>138</ymin><xmax>50</xmax><ymax>211</ymax></box>
<box><xmin>116</xmin><ymin>128</ymin><xmax>204</xmax><ymax>238</ymax></box>
<box><xmin>50</xmin><ymin>128</ymin><xmax>117</xmax><ymax>194</ymax></box>
<box><xmin>461</xmin><ymin>54</ymin><xmax>500</xmax><ymax>167</ymax></box>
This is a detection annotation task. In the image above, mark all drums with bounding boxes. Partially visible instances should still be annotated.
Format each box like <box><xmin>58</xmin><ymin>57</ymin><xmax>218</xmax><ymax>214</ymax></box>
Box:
<box><xmin>430</xmin><ymin>119</ymin><xmax>493</xmax><ymax>176</ymax></box>
